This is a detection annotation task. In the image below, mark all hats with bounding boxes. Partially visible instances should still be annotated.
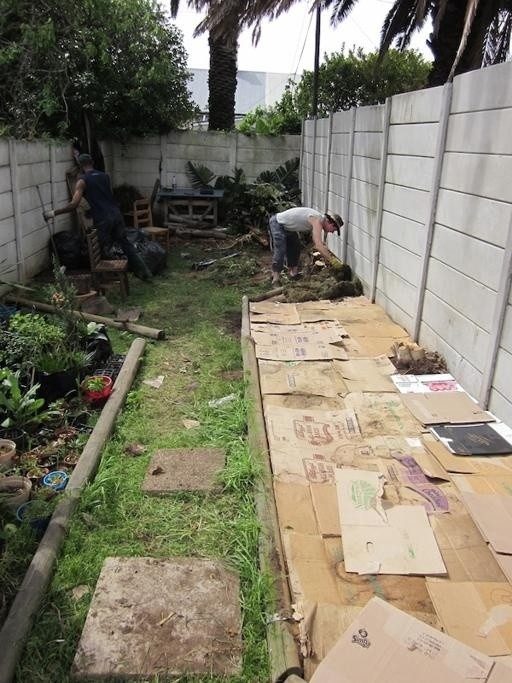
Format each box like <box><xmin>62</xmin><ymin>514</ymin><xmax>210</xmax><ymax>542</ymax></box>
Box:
<box><xmin>324</xmin><ymin>212</ymin><xmax>344</xmax><ymax>236</ymax></box>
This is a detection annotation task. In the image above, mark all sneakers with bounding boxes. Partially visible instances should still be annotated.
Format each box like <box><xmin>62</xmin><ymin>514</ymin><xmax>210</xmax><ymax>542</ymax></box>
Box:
<box><xmin>271</xmin><ymin>271</ymin><xmax>281</xmax><ymax>285</ymax></box>
<box><xmin>288</xmin><ymin>266</ymin><xmax>298</xmax><ymax>278</ymax></box>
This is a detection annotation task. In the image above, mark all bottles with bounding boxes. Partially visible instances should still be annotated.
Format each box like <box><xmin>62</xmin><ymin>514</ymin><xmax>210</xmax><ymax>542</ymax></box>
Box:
<box><xmin>172</xmin><ymin>176</ymin><xmax>177</xmax><ymax>191</ymax></box>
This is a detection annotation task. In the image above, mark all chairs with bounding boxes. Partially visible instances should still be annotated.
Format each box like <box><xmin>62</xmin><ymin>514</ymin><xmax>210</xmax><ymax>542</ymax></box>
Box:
<box><xmin>85</xmin><ymin>228</ymin><xmax>129</xmax><ymax>298</ymax></box>
<box><xmin>134</xmin><ymin>197</ymin><xmax>169</xmax><ymax>254</ymax></box>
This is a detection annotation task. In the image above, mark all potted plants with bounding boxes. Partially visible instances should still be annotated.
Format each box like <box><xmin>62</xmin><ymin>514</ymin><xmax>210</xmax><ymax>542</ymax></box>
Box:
<box><xmin>0</xmin><ymin>305</ymin><xmax>115</xmax><ymax>526</ymax></box>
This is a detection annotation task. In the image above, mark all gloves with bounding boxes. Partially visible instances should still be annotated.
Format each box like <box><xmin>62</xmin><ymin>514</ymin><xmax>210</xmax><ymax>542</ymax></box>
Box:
<box><xmin>42</xmin><ymin>209</ymin><xmax>55</xmax><ymax>219</ymax></box>
<box><xmin>329</xmin><ymin>257</ymin><xmax>344</xmax><ymax>269</ymax></box>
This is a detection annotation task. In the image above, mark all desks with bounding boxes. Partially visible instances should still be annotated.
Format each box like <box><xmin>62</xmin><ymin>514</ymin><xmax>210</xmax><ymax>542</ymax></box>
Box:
<box><xmin>156</xmin><ymin>189</ymin><xmax>226</xmax><ymax>228</ymax></box>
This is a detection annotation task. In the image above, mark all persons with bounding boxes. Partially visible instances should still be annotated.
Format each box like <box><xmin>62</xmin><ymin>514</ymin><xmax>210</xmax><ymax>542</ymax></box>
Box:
<box><xmin>269</xmin><ymin>206</ymin><xmax>344</xmax><ymax>291</ymax></box>
<box><xmin>42</xmin><ymin>148</ymin><xmax>154</xmax><ymax>282</ymax></box>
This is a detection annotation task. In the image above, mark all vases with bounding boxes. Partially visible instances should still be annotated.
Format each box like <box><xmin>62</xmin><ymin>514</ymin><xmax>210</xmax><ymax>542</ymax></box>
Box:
<box><xmin>68</xmin><ymin>274</ymin><xmax>91</xmax><ymax>295</ymax></box>
